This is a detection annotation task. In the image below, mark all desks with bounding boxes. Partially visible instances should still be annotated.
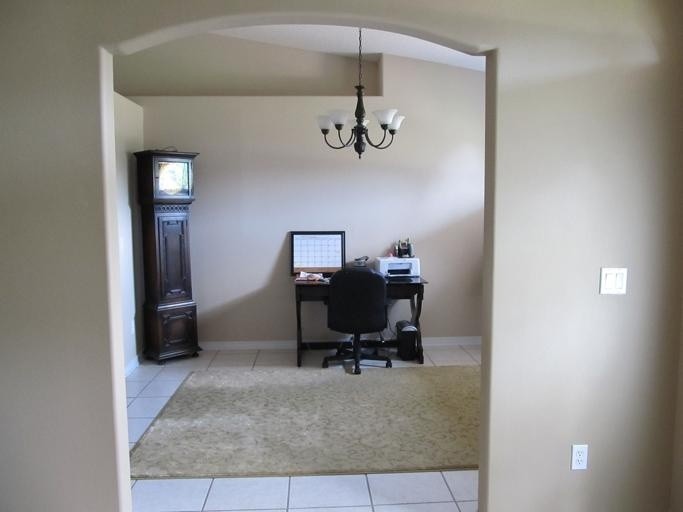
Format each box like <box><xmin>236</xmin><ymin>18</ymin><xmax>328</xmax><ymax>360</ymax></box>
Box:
<box><xmin>295</xmin><ymin>273</ymin><xmax>428</xmax><ymax>366</ymax></box>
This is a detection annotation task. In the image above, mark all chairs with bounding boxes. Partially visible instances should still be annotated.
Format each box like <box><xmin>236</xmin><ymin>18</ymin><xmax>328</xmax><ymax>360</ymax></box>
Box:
<box><xmin>322</xmin><ymin>267</ymin><xmax>393</xmax><ymax>375</ymax></box>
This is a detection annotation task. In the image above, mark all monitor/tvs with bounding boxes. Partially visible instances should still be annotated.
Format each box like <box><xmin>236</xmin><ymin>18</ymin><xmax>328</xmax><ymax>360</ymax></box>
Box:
<box><xmin>288</xmin><ymin>230</ymin><xmax>346</xmax><ymax>278</ymax></box>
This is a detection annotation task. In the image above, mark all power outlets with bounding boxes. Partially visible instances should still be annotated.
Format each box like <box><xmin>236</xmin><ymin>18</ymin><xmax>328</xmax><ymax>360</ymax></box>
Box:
<box><xmin>571</xmin><ymin>445</ymin><xmax>589</xmax><ymax>470</ymax></box>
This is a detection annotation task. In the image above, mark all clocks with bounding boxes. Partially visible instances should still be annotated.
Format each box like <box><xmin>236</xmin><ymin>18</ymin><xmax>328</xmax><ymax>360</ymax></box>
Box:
<box><xmin>131</xmin><ymin>147</ymin><xmax>203</xmax><ymax>366</ymax></box>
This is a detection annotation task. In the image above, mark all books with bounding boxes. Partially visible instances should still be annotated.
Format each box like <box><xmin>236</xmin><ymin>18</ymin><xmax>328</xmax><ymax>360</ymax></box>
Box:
<box><xmin>295</xmin><ymin>270</ymin><xmax>324</xmax><ymax>281</ymax></box>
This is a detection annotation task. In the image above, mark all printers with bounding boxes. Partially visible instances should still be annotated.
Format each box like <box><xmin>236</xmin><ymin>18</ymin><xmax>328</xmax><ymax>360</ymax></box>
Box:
<box><xmin>374</xmin><ymin>256</ymin><xmax>421</xmax><ymax>280</ymax></box>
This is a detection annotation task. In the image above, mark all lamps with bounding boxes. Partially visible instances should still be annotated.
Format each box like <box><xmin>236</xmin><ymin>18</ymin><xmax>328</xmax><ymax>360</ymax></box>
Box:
<box><xmin>314</xmin><ymin>25</ymin><xmax>406</xmax><ymax>159</ymax></box>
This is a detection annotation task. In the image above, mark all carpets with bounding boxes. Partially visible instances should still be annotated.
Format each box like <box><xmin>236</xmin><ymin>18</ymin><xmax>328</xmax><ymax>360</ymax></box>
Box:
<box><xmin>130</xmin><ymin>363</ymin><xmax>482</xmax><ymax>480</ymax></box>
